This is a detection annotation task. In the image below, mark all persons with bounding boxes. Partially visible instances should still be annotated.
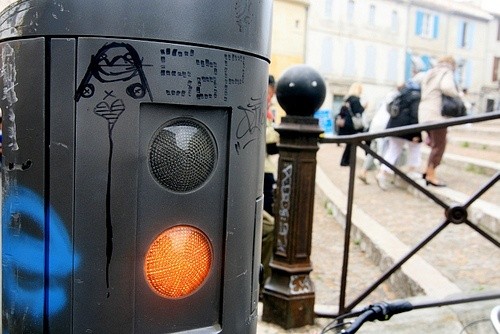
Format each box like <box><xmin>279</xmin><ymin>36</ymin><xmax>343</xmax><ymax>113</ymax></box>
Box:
<box><xmin>263</xmin><ymin>75</ymin><xmax>286</xmax><ymax>216</ymax></box>
<box><xmin>418</xmin><ymin>56</ymin><xmax>464</xmax><ymax>188</ymax></box>
<box><xmin>357</xmin><ymin>71</ymin><xmax>427</xmax><ymax>191</ymax></box>
<box><xmin>336</xmin><ymin>82</ymin><xmax>369</xmax><ymax>167</ymax></box>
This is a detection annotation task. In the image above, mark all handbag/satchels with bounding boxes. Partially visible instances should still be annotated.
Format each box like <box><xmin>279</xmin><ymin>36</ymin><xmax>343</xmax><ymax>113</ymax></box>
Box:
<box><xmin>346</xmin><ymin>103</ymin><xmax>364</xmax><ymax>130</ymax></box>
<box><xmin>439</xmin><ymin>70</ymin><xmax>468</xmax><ymax>117</ymax></box>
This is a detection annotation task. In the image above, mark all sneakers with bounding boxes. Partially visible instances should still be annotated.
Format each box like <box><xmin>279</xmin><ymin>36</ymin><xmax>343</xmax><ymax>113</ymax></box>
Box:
<box><xmin>376</xmin><ymin>174</ymin><xmax>386</xmax><ymax>190</ymax></box>
<box><xmin>407</xmin><ymin>171</ymin><xmax>422</xmax><ymax>179</ymax></box>
<box><xmin>358</xmin><ymin>173</ymin><xmax>371</xmax><ymax>186</ymax></box>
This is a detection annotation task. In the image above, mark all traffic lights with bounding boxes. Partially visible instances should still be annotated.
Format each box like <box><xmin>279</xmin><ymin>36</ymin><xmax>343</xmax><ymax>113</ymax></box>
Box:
<box><xmin>133</xmin><ymin>102</ymin><xmax>232</xmax><ymax>334</ymax></box>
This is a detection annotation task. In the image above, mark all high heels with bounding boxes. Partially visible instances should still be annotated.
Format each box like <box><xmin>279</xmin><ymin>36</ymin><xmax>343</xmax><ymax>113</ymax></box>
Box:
<box><xmin>423</xmin><ymin>173</ymin><xmax>447</xmax><ymax>188</ymax></box>
